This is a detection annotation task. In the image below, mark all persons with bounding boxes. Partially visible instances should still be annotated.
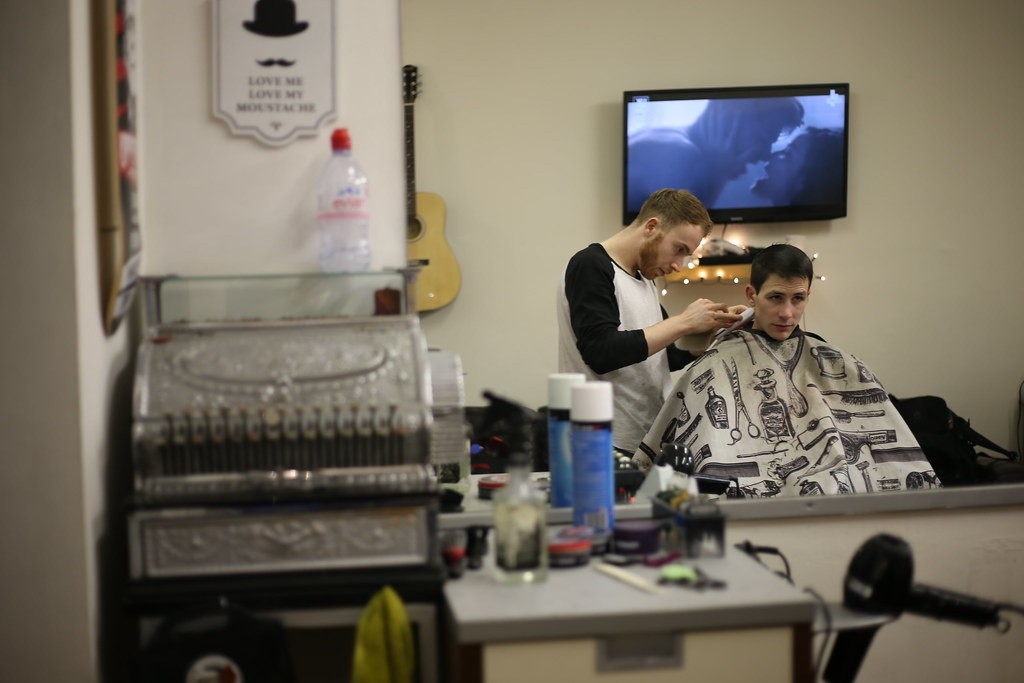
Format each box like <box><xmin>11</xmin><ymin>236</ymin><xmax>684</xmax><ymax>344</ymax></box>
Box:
<box><xmin>557</xmin><ymin>187</ymin><xmax>747</xmax><ymax>457</ymax></box>
<box><xmin>627</xmin><ymin>97</ymin><xmax>804</xmax><ymax>211</ymax></box>
<box><xmin>697</xmin><ymin>108</ymin><xmax>845</xmax><ymax>206</ymax></box>
<box><xmin>682</xmin><ymin>243</ymin><xmax>888</xmax><ymax>501</ymax></box>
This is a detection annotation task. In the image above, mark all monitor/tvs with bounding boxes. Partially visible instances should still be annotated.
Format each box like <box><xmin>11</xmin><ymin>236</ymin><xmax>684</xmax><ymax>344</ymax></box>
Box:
<box><xmin>623</xmin><ymin>82</ymin><xmax>849</xmax><ymax>226</ymax></box>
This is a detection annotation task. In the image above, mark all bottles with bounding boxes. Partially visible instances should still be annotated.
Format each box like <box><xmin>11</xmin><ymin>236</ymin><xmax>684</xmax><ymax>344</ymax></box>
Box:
<box><xmin>472</xmin><ymin>391</ymin><xmax>548</xmax><ymax>586</ymax></box>
<box><xmin>312</xmin><ymin>128</ymin><xmax>372</xmax><ymax>275</ymax></box>
<box><xmin>546</xmin><ymin>374</ymin><xmax>587</xmax><ymax>510</ymax></box>
<box><xmin>568</xmin><ymin>381</ymin><xmax>615</xmax><ymax>547</ymax></box>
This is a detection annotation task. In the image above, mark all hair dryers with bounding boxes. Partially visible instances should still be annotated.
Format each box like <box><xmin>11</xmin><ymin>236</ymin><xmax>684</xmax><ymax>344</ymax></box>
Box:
<box><xmin>821</xmin><ymin>532</ymin><xmax>1011</xmax><ymax>682</ymax></box>
<box><xmin>659</xmin><ymin>444</ymin><xmax>734</xmax><ymax>495</ymax></box>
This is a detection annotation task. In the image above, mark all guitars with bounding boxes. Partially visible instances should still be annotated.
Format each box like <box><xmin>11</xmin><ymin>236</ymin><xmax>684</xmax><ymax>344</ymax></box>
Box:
<box><xmin>401</xmin><ymin>65</ymin><xmax>462</xmax><ymax>312</ymax></box>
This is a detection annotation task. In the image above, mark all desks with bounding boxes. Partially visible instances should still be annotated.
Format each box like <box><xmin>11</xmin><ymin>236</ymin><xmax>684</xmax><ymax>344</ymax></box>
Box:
<box><xmin>441</xmin><ymin>530</ymin><xmax>816</xmax><ymax>683</ymax></box>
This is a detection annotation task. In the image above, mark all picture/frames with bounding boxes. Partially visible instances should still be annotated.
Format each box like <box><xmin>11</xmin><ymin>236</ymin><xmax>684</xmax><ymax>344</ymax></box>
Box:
<box><xmin>214</xmin><ymin>0</ymin><xmax>340</xmax><ymax>148</ymax></box>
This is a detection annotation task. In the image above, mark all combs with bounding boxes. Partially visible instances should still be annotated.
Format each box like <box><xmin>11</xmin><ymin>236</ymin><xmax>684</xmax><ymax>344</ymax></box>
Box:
<box><xmin>595</xmin><ymin>561</ymin><xmax>656</xmax><ymax>594</ymax></box>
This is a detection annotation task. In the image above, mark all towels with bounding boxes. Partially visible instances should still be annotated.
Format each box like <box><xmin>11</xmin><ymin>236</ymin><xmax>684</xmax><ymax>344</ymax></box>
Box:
<box><xmin>354</xmin><ymin>584</ymin><xmax>416</xmax><ymax>683</ymax></box>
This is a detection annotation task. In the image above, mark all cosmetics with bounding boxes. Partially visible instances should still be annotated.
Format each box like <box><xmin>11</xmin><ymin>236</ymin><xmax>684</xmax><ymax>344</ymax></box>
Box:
<box><xmin>433</xmin><ymin>423</ymin><xmax>471</xmax><ymax>495</ymax></box>
<box><xmin>490</xmin><ymin>458</ymin><xmax>548</xmax><ymax>583</ymax></box>
<box><xmin>547</xmin><ymin>538</ymin><xmax>591</xmax><ymax>569</ymax></box>
<box><xmin>613</xmin><ymin>521</ymin><xmax>661</xmax><ymax>553</ymax></box>
<box><xmin>557</xmin><ymin>524</ymin><xmax>609</xmax><ymax>555</ymax></box>
<box><xmin>479</xmin><ymin>475</ymin><xmax>508</xmax><ymax>499</ymax></box>
<box><xmin>572</xmin><ymin>381</ymin><xmax>614</xmax><ymax>532</ymax></box>
<box><xmin>549</xmin><ymin>372</ymin><xmax>585</xmax><ymax>506</ymax></box>
